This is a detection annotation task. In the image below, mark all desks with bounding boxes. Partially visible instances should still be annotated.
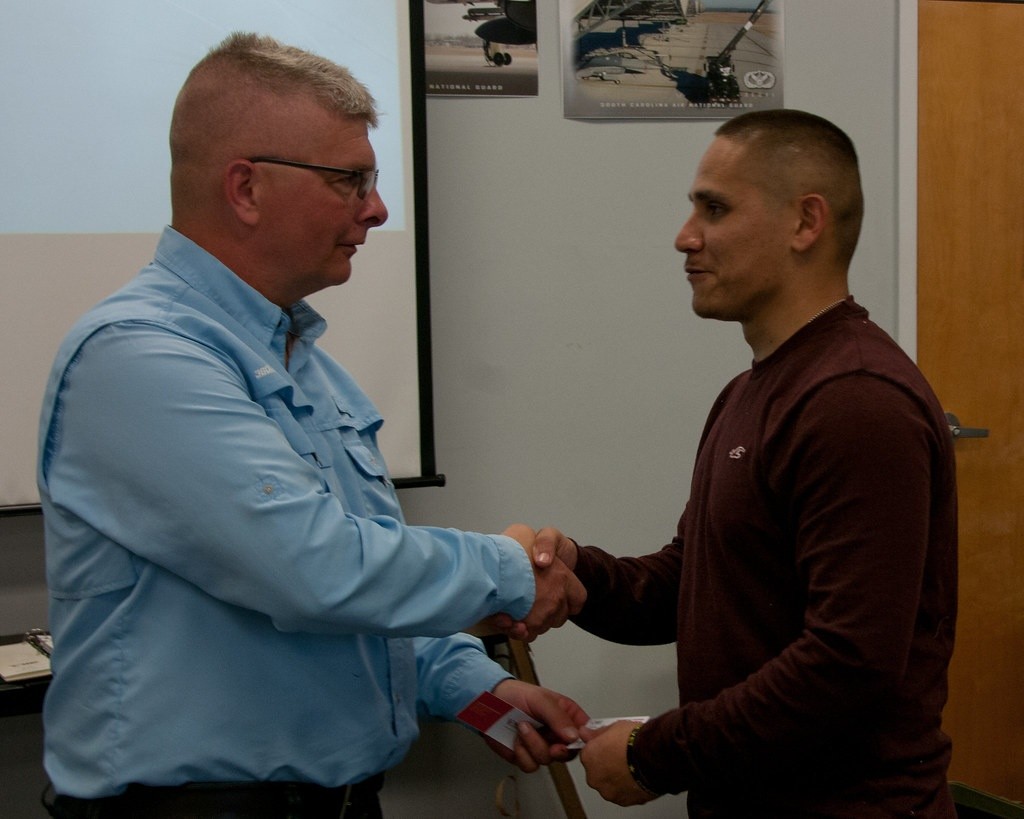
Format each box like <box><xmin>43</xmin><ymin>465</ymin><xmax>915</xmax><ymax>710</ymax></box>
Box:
<box><xmin>0</xmin><ymin>630</ymin><xmax>54</xmax><ymax>717</ymax></box>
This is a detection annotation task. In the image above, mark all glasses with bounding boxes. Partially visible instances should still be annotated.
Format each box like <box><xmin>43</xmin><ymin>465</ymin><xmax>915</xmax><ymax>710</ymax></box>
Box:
<box><xmin>245</xmin><ymin>148</ymin><xmax>385</xmax><ymax>196</ymax></box>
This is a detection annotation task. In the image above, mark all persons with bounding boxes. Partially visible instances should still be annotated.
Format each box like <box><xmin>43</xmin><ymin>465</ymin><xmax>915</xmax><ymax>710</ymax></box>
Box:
<box><xmin>35</xmin><ymin>32</ymin><xmax>590</xmax><ymax>819</ymax></box>
<box><xmin>491</xmin><ymin>110</ymin><xmax>960</xmax><ymax>819</ymax></box>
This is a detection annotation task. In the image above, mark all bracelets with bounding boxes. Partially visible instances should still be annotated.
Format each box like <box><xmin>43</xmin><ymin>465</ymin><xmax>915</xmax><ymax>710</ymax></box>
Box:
<box><xmin>625</xmin><ymin>727</ymin><xmax>662</xmax><ymax>797</ymax></box>
